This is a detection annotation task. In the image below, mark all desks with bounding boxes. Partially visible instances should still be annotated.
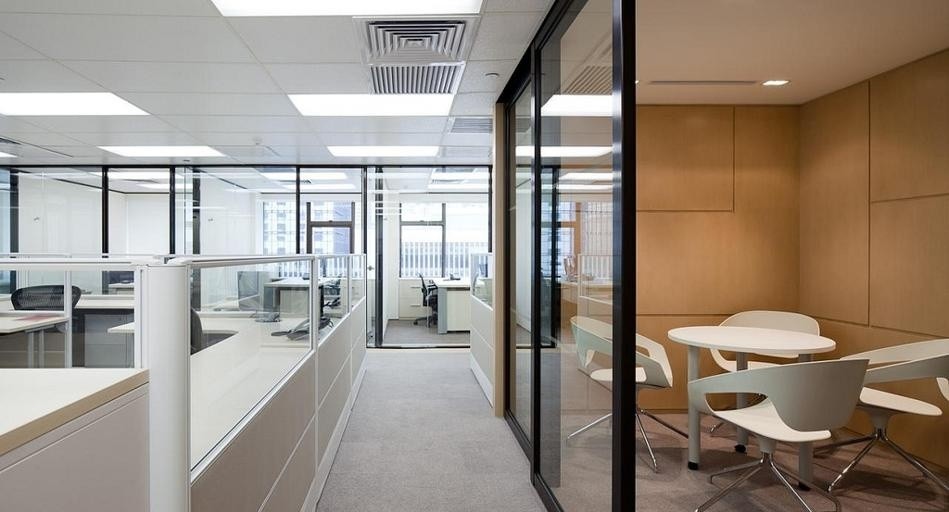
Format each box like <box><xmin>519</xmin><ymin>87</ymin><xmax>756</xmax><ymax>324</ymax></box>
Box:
<box><xmin>432</xmin><ymin>277</ymin><xmax>471</xmax><ymax>334</ymax></box>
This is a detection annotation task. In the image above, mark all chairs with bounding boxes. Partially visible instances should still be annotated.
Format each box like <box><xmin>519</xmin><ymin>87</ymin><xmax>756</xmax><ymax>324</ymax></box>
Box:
<box><xmin>413</xmin><ymin>273</ymin><xmax>438</xmax><ymax>328</ymax></box>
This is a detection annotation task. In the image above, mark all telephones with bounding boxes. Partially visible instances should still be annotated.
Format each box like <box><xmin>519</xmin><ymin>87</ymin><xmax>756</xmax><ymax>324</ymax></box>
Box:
<box><xmin>286</xmin><ymin>315</ymin><xmax>330</xmax><ymax>341</ymax></box>
